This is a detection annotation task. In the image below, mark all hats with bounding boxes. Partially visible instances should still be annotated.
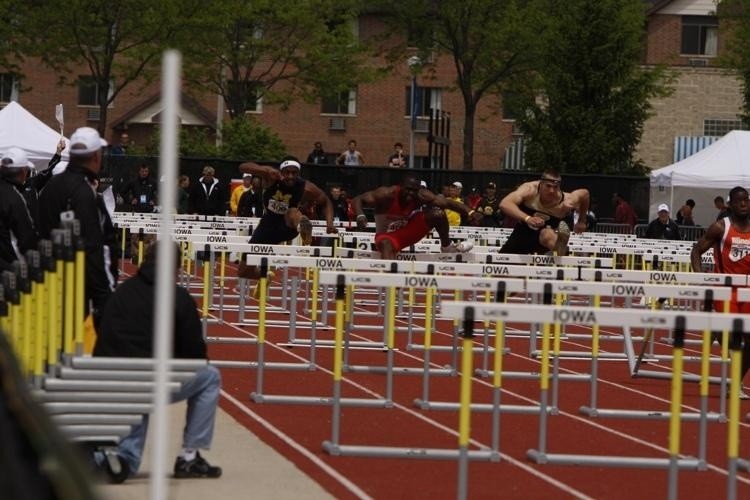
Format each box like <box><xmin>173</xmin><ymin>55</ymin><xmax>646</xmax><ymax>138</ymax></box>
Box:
<box><xmin>2</xmin><ymin>149</ymin><xmax>35</xmax><ymax>169</ymax></box>
<box><xmin>657</xmin><ymin>204</ymin><xmax>669</xmax><ymax>213</ymax></box>
<box><xmin>420</xmin><ymin>181</ymin><xmax>426</xmax><ymax>187</ymax></box>
<box><xmin>453</xmin><ymin>182</ymin><xmax>463</xmax><ymax>188</ymax></box>
<box><xmin>68</xmin><ymin>127</ymin><xmax>108</xmax><ymax>154</ymax></box>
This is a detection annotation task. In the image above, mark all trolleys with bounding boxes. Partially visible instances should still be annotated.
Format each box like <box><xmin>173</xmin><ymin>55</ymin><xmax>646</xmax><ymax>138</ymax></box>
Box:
<box><xmin>1</xmin><ymin>217</ymin><xmax>210</xmax><ymax>480</ymax></box>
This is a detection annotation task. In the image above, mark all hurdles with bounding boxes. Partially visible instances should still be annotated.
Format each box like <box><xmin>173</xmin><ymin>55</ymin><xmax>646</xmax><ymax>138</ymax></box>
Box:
<box><xmin>318</xmin><ymin>269</ymin><xmax>525</xmax><ymax>464</ymax></box>
<box><xmin>190</xmin><ymin>241</ymin><xmax>333</xmax><ymax>371</ymax></box>
<box><xmin>112</xmin><ymin>213</ymin><xmax>518</xmax><ymax>355</ymax></box>
<box><xmin>244</xmin><ymin>253</ymin><xmax>413</xmax><ymax>409</ymax></box>
<box><xmin>462</xmin><ymin>253</ymin><xmax>615</xmax><ymax>382</ymax></box>
<box><xmin>566</xmin><ymin>231</ymin><xmax>734</xmax><ymax>382</ymax></box>
<box><xmin>413</xmin><ymin>259</ymin><xmax>579</xmax><ymax>417</ymax></box>
<box><xmin>441</xmin><ymin>301</ymin><xmax>711</xmax><ymax>499</ymax></box>
<box><xmin>579</xmin><ymin>268</ymin><xmax>747</xmax><ymax>423</ymax></box>
<box><xmin>334</xmin><ymin>247</ymin><xmax>461</xmax><ymax>377</ymax></box>
<box><xmin>710</xmin><ymin>313</ymin><xmax>750</xmax><ymax>500</ymax></box>
<box><xmin>736</xmin><ymin>287</ymin><xmax>748</xmax><ymax>474</ymax></box>
<box><xmin>525</xmin><ymin>278</ymin><xmax>732</xmax><ymax>472</ymax></box>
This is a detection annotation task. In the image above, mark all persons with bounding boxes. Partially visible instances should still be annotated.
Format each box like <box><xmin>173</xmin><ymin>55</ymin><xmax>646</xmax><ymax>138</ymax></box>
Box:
<box><xmin>352</xmin><ymin>175</ymin><xmax>483</xmax><ymax>298</ymax></box>
<box><xmin>237</xmin><ymin>155</ymin><xmax>335</xmax><ymax>300</ymax></box>
<box><xmin>229</xmin><ymin>171</ymin><xmax>597</xmax><ymax>234</ymax></box>
<box><xmin>611</xmin><ymin>185</ymin><xmax>749</xmax><ymax>401</ymax></box>
<box><xmin>498</xmin><ymin>168</ymin><xmax>590</xmax><ymax>297</ymax></box>
<box><xmin>3</xmin><ymin>127</ymin><xmax>223</xmax><ymax>478</ymax></box>
<box><xmin>334</xmin><ymin>140</ymin><xmax>368</xmax><ymax>166</ymax></box>
<box><xmin>386</xmin><ymin>143</ymin><xmax>409</xmax><ymax>168</ymax></box>
<box><xmin>306</xmin><ymin>140</ymin><xmax>328</xmax><ymax>164</ymax></box>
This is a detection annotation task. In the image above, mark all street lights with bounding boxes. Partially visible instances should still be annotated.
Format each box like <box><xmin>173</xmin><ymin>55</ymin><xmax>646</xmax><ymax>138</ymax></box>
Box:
<box><xmin>406</xmin><ymin>56</ymin><xmax>424</xmax><ymax>171</ymax></box>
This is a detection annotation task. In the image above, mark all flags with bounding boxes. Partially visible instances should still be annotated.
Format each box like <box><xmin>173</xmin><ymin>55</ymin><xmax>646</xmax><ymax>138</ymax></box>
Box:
<box><xmin>410</xmin><ymin>77</ymin><xmax>422</xmax><ymax>131</ymax></box>
<box><xmin>56</xmin><ymin>104</ymin><xmax>64</xmax><ymax>128</ymax></box>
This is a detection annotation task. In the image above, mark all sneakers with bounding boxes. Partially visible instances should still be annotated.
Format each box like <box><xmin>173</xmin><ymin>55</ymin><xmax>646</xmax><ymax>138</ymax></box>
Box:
<box><xmin>299</xmin><ymin>215</ymin><xmax>313</xmax><ymax>244</ymax></box>
<box><xmin>253</xmin><ymin>272</ymin><xmax>274</xmax><ymax>297</ymax></box>
<box><xmin>174</xmin><ymin>450</ymin><xmax>221</xmax><ymax>478</ymax></box>
<box><xmin>441</xmin><ymin>239</ymin><xmax>473</xmax><ymax>253</ymax></box>
<box><xmin>555</xmin><ymin>222</ymin><xmax>571</xmax><ymax>256</ymax></box>
